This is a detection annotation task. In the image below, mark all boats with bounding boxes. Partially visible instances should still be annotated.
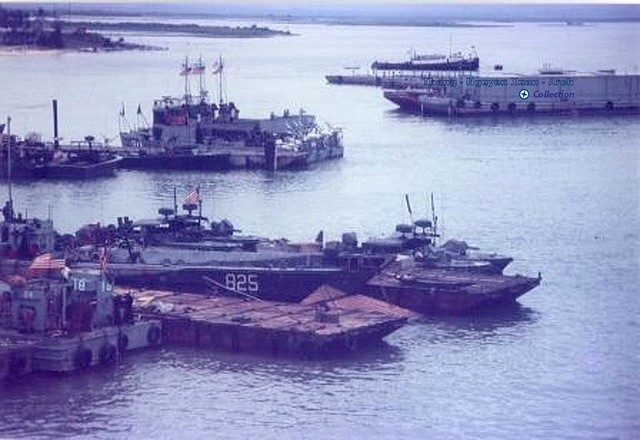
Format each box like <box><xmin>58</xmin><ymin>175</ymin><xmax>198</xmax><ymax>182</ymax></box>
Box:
<box><xmin>0</xmin><ymin>16</ymin><xmax>110</xmax><ymax>49</ymax></box>
<box><xmin>0</xmin><ymin>252</ymin><xmax>162</xmax><ymax>379</ymax></box>
<box><xmin>365</xmin><ymin>268</ymin><xmax>543</xmax><ymax>314</ymax></box>
<box><xmin>0</xmin><ymin>263</ymin><xmax>492</xmax><ymax>301</ymax></box>
<box><xmin>1</xmin><ymin>186</ymin><xmax>512</xmax><ymax>265</ymax></box>
<box><xmin>325</xmin><ymin>66</ymin><xmax>480</xmax><ymax>84</ymax></box>
<box><xmin>373</xmin><ymin>56</ymin><xmax>479</xmax><ymax>70</ymax></box>
<box><xmin>0</xmin><ymin>99</ymin><xmax>123</xmax><ymax>180</ymax></box>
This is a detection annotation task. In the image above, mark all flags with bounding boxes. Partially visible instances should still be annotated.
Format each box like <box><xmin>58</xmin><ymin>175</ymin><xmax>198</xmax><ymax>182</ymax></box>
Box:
<box><xmin>179</xmin><ymin>63</ymin><xmax>191</xmax><ymax>77</ymax></box>
<box><xmin>210</xmin><ymin>59</ymin><xmax>222</xmax><ymax>74</ymax></box>
<box><xmin>29</xmin><ymin>250</ymin><xmax>68</xmax><ymax>269</ymax></box>
<box><xmin>182</xmin><ymin>190</ymin><xmax>202</xmax><ymax>208</ymax></box>
<box><xmin>192</xmin><ymin>61</ymin><xmax>206</xmax><ymax>75</ymax></box>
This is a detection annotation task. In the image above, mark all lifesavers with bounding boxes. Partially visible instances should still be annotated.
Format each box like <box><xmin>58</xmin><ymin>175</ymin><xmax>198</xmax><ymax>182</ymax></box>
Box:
<box><xmin>99</xmin><ymin>345</ymin><xmax>116</xmax><ymax>364</ymax></box>
<box><xmin>73</xmin><ymin>345</ymin><xmax>93</xmax><ymax>369</ymax></box>
<box><xmin>119</xmin><ymin>335</ymin><xmax>129</xmax><ymax>351</ymax></box>
<box><xmin>147</xmin><ymin>326</ymin><xmax>160</xmax><ymax>343</ymax></box>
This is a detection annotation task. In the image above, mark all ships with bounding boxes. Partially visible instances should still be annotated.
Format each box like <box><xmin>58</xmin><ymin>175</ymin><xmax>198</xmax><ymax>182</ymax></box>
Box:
<box><xmin>71</xmin><ymin>56</ymin><xmax>344</xmax><ymax>169</ymax></box>
<box><xmin>383</xmin><ymin>65</ymin><xmax>640</xmax><ymax>115</ymax></box>
